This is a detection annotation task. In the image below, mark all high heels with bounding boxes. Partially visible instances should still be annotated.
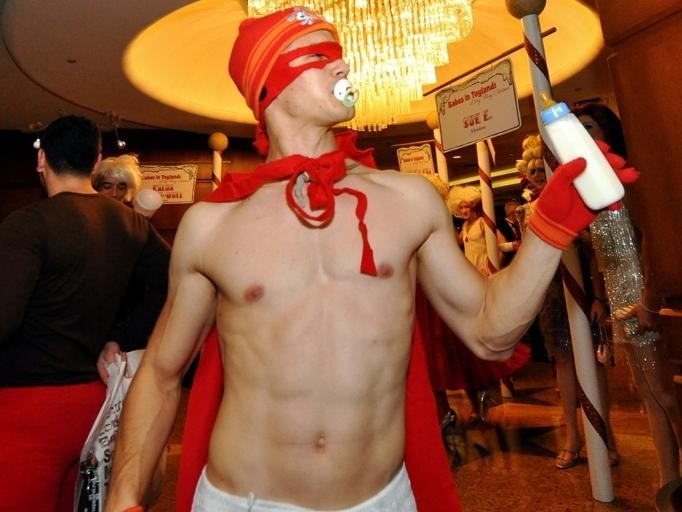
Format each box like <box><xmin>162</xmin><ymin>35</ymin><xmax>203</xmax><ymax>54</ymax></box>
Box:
<box><xmin>440</xmin><ymin>408</ymin><xmax>457</xmax><ymax>440</ymax></box>
<box><xmin>469</xmin><ymin>390</ymin><xmax>498</xmax><ymax>426</ymax></box>
<box><xmin>555</xmin><ymin>431</ymin><xmax>585</xmax><ymax>469</ymax></box>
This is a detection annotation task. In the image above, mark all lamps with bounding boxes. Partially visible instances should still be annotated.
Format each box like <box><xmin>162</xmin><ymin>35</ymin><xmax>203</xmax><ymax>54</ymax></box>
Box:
<box><xmin>245</xmin><ymin>0</ymin><xmax>478</xmax><ymax>133</ymax></box>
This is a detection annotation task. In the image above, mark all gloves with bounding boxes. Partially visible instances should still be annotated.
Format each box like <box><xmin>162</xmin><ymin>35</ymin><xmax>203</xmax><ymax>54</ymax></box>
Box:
<box><xmin>523</xmin><ymin>138</ymin><xmax>642</xmax><ymax>253</ymax></box>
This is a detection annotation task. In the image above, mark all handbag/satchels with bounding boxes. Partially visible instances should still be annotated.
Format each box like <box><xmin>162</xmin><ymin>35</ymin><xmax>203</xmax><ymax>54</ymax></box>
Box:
<box><xmin>596</xmin><ymin>318</ymin><xmax>612</xmax><ymax>364</ymax></box>
<box><xmin>73</xmin><ymin>347</ymin><xmax>170</xmax><ymax>511</ymax></box>
<box><xmin>614</xmin><ymin>300</ymin><xmax>641</xmax><ymax>339</ymax></box>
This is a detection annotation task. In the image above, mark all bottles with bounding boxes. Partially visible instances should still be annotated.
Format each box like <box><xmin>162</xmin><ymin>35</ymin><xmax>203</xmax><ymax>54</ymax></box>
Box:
<box><xmin>539</xmin><ymin>90</ymin><xmax>626</xmax><ymax>211</ymax></box>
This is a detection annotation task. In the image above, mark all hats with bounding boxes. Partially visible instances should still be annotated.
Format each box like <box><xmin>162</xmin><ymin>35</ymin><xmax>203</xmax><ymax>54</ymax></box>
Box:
<box><xmin>227</xmin><ymin>5</ymin><xmax>340</xmax><ymax>123</ymax></box>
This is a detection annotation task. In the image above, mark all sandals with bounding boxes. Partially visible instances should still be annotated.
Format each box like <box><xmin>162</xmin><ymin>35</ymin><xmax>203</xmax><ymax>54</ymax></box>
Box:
<box><xmin>606</xmin><ymin>431</ymin><xmax>619</xmax><ymax>466</ymax></box>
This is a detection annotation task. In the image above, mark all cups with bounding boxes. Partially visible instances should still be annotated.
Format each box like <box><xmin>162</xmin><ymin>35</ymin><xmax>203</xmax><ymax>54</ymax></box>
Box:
<box><xmin>132</xmin><ymin>188</ymin><xmax>164</xmax><ymax>220</ymax></box>
